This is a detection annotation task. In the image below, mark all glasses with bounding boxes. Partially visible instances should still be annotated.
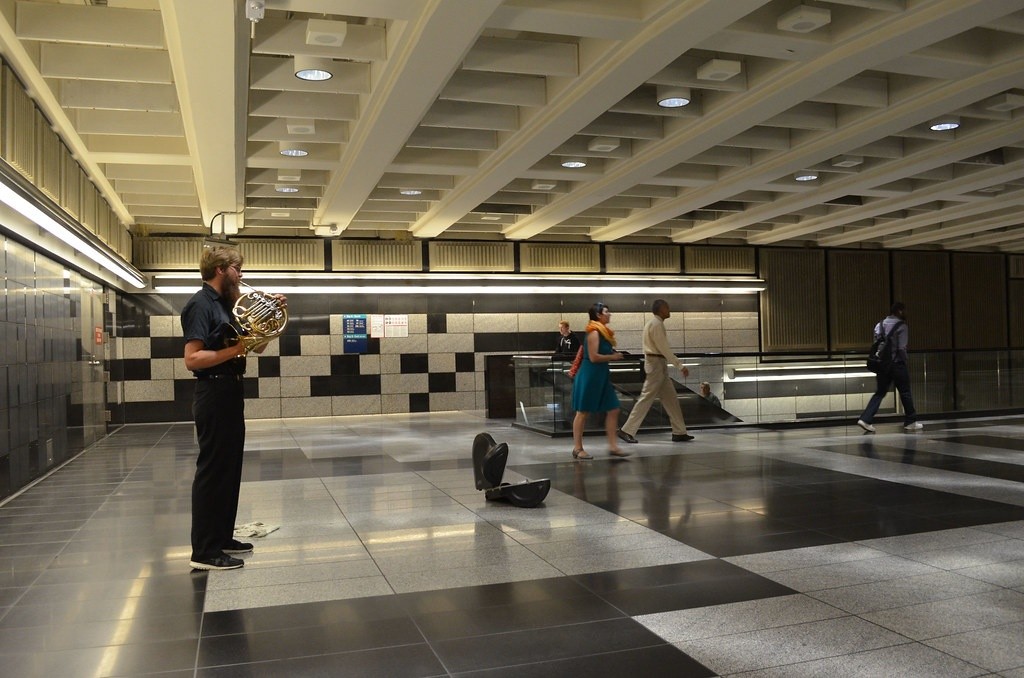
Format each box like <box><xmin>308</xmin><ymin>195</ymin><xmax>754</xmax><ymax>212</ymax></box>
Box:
<box><xmin>231</xmin><ymin>266</ymin><xmax>241</xmax><ymax>272</ymax></box>
<box><xmin>598</xmin><ymin>303</ymin><xmax>603</xmax><ymax>313</ymax></box>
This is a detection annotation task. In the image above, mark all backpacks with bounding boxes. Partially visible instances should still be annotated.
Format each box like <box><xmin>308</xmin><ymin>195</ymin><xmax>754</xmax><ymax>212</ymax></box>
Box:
<box><xmin>866</xmin><ymin>318</ymin><xmax>906</xmax><ymax>376</ymax></box>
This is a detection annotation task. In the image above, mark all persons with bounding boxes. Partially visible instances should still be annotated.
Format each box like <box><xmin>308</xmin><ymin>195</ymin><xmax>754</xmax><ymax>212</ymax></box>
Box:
<box><xmin>571</xmin><ymin>302</ymin><xmax>633</xmax><ymax>459</ymax></box>
<box><xmin>556</xmin><ymin>321</ymin><xmax>580</xmax><ymax>353</ymax></box>
<box><xmin>701</xmin><ymin>382</ymin><xmax>721</xmax><ymax>408</ymax></box>
<box><xmin>618</xmin><ymin>299</ymin><xmax>694</xmax><ymax>442</ymax></box>
<box><xmin>180</xmin><ymin>246</ymin><xmax>288</xmax><ymax>569</ymax></box>
<box><xmin>857</xmin><ymin>302</ymin><xmax>923</xmax><ymax>431</ymax></box>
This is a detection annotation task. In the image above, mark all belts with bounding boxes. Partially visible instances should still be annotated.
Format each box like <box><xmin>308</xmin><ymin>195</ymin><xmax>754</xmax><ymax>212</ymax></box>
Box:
<box><xmin>198</xmin><ymin>373</ymin><xmax>242</xmax><ymax>381</ymax></box>
<box><xmin>646</xmin><ymin>354</ymin><xmax>665</xmax><ymax>358</ymax></box>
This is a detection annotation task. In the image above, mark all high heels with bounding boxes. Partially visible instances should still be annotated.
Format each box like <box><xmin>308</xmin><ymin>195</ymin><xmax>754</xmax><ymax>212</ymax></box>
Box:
<box><xmin>610</xmin><ymin>448</ymin><xmax>632</xmax><ymax>456</ymax></box>
<box><xmin>572</xmin><ymin>447</ymin><xmax>593</xmax><ymax>459</ymax></box>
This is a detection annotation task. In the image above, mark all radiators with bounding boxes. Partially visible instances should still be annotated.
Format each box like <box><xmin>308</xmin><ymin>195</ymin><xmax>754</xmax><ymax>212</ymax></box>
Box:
<box><xmin>893</xmin><ymin>251</ymin><xmax>953</xmax><ymax>351</ymax></box>
<box><xmin>954</xmin><ymin>252</ymin><xmax>1007</xmax><ymax>349</ymax></box>
<box><xmin>1009</xmin><ymin>255</ymin><xmax>1024</xmax><ymax>279</ymax></box>
<box><xmin>332</xmin><ymin>240</ymin><xmax>422</xmax><ymax>271</ymax></box>
<box><xmin>758</xmin><ymin>248</ymin><xmax>828</xmax><ymax>352</ymax></box>
<box><xmin>954</xmin><ymin>351</ymin><xmax>1010</xmax><ymax>411</ymax></box>
<box><xmin>1008</xmin><ymin>280</ymin><xmax>1024</xmax><ymax>348</ymax></box>
<box><xmin>828</xmin><ymin>250</ymin><xmax>892</xmax><ymax>353</ymax></box>
<box><xmin>520</xmin><ymin>243</ymin><xmax>600</xmax><ymax>273</ymax></box>
<box><xmin>605</xmin><ymin>245</ymin><xmax>681</xmax><ymax>273</ymax></box>
<box><xmin>684</xmin><ymin>246</ymin><xmax>755</xmax><ymax>274</ymax></box>
<box><xmin>133</xmin><ymin>236</ymin><xmax>324</xmax><ymax>270</ymax></box>
<box><xmin>895</xmin><ymin>353</ymin><xmax>950</xmax><ymax>415</ymax></box>
<box><xmin>0</xmin><ymin>57</ymin><xmax>133</xmax><ymax>263</ymax></box>
<box><xmin>429</xmin><ymin>241</ymin><xmax>515</xmax><ymax>271</ymax></box>
<box><xmin>1010</xmin><ymin>350</ymin><xmax>1024</xmax><ymax>408</ymax></box>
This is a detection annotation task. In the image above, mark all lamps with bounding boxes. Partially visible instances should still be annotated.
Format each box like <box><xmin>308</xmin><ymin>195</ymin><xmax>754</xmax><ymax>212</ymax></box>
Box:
<box><xmin>928</xmin><ymin>116</ymin><xmax>960</xmax><ymax>132</ymax></box>
<box><xmin>561</xmin><ymin>156</ymin><xmax>588</xmax><ymax>168</ymax></box>
<box><xmin>657</xmin><ymin>86</ymin><xmax>691</xmax><ymax>108</ymax></box>
<box><xmin>795</xmin><ymin>171</ymin><xmax>818</xmax><ymax>182</ymax></box>
<box><xmin>279</xmin><ymin>142</ymin><xmax>309</xmax><ymax>157</ymax></box>
<box><xmin>294</xmin><ymin>56</ymin><xmax>334</xmax><ymax>81</ymax></box>
<box><xmin>275</xmin><ymin>186</ymin><xmax>299</xmax><ymax>193</ymax></box>
<box><xmin>400</xmin><ymin>189</ymin><xmax>422</xmax><ymax>195</ymax></box>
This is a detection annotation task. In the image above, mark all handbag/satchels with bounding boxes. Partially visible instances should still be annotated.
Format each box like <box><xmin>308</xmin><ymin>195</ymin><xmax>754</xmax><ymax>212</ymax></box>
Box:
<box><xmin>567</xmin><ymin>345</ymin><xmax>584</xmax><ymax>378</ymax></box>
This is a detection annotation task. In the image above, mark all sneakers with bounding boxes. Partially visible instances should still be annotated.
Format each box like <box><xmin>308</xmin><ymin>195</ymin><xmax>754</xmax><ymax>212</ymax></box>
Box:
<box><xmin>189</xmin><ymin>550</ymin><xmax>244</xmax><ymax>569</ymax></box>
<box><xmin>618</xmin><ymin>427</ymin><xmax>638</xmax><ymax>443</ymax></box>
<box><xmin>221</xmin><ymin>539</ymin><xmax>253</xmax><ymax>553</ymax></box>
<box><xmin>903</xmin><ymin>421</ymin><xmax>924</xmax><ymax>429</ymax></box>
<box><xmin>858</xmin><ymin>419</ymin><xmax>875</xmax><ymax>431</ymax></box>
<box><xmin>672</xmin><ymin>434</ymin><xmax>694</xmax><ymax>441</ymax></box>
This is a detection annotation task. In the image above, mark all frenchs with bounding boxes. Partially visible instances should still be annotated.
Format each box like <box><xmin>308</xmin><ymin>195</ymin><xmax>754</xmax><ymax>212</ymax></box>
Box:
<box><xmin>219</xmin><ymin>280</ymin><xmax>288</xmax><ymax>375</ymax></box>
